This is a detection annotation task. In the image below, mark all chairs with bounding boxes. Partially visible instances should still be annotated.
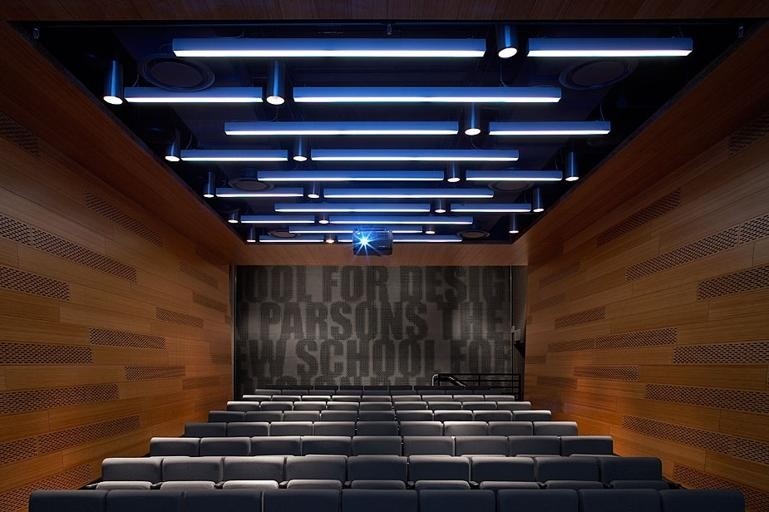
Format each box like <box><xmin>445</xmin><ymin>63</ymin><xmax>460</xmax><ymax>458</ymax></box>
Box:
<box><xmin>27</xmin><ymin>384</ymin><xmax>747</xmax><ymax>511</ymax></box>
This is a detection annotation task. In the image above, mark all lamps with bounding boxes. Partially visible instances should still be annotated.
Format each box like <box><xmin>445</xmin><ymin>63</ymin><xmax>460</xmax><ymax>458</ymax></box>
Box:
<box><xmin>100</xmin><ymin>27</ymin><xmax>694</xmax><ymax>248</ymax></box>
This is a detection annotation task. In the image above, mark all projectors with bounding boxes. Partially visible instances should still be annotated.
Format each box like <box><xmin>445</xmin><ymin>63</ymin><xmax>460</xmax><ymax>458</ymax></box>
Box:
<box><xmin>353</xmin><ymin>232</ymin><xmax>393</xmax><ymax>256</ymax></box>
